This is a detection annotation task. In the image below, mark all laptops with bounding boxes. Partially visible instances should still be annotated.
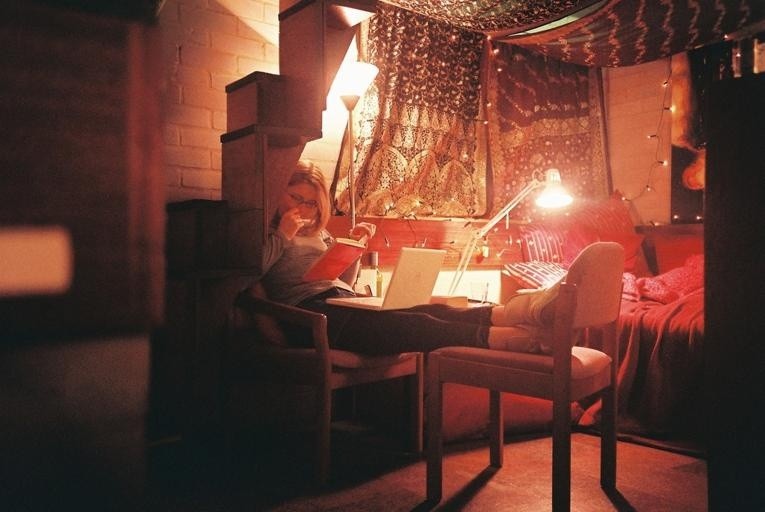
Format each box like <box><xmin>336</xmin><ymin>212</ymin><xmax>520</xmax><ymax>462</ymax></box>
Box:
<box><xmin>325</xmin><ymin>247</ymin><xmax>449</xmax><ymax>310</ymax></box>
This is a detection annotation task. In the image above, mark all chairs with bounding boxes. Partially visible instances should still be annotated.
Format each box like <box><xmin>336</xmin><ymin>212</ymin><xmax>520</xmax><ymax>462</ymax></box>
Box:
<box><xmin>426</xmin><ymin>242</ymin><xmax>625</xmax><ymax>511</ymax></box>
<box><xmin>227</xmin><ymin>283</ymin><xmax>421</xmax><ymax>482</ymax></box>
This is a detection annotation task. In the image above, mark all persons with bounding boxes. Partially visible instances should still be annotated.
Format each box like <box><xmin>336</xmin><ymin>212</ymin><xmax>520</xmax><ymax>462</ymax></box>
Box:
<box><xmin>260</xmin><ymin>159</ymin><xmax>580</xmax><ymax>356</ymax></box>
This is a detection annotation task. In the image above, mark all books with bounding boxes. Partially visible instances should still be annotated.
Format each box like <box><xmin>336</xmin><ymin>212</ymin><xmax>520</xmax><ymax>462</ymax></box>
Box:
<box><xmin>302</xmin><ymin>232</ymin><xmax>373</xmax><ymax>280</ymax></box>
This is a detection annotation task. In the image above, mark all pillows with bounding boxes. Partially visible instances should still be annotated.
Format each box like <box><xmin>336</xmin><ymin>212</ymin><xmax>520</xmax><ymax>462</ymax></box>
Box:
<box><xmin>523</xmin><ymin>192</ymin><xmax>653</xmax><ymax>281</ymax></box>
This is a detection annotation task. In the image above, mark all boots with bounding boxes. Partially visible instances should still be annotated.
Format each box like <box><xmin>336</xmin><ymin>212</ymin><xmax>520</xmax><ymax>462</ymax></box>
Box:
<box><xmin>501</xmin><ymin>271</ymin><xmax>567</xmax><ymax>327</ymax></box>
<box><xmin>503</xmin><ymin>323</ymin><xmax>580</xmax><ymax>358</ymax></box>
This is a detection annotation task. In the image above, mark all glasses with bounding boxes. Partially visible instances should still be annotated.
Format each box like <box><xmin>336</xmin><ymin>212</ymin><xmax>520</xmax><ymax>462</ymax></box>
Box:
<box><xmin>283</xmin><ymin>191</ymin><xmax>319</xmax><ymax>210</ymax></box>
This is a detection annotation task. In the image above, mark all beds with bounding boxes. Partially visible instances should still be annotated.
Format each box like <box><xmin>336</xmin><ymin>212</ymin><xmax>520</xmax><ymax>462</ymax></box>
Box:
<box><xmin>500</xmin><ymin>217</ymin><xmax>706</xmax><ymax>452</ymax></box>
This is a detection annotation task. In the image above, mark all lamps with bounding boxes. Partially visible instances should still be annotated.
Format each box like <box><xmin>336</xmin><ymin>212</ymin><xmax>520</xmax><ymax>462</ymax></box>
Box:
<box><xmin>448</xmin><ymin>168</ymin><xmax>573</xmax><ymax>296</ymax></box>
<box><xmin>334</xmin><ymin>61</ymin><xmax>380</xmax><ymax>239</ymax></box>
